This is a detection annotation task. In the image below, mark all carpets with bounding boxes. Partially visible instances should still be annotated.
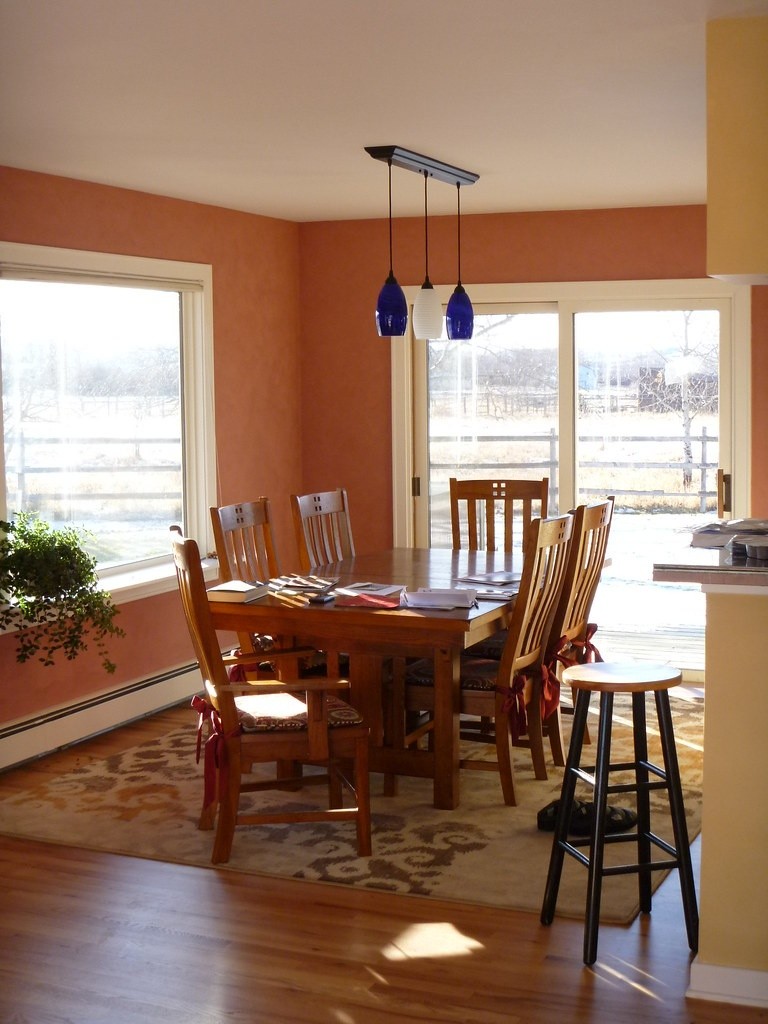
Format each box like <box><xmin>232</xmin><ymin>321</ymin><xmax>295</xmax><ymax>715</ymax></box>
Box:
<box><xmin>1</xmin><ymin>684</ymin><xmax>704</xmax><ymax>929</ymax></box>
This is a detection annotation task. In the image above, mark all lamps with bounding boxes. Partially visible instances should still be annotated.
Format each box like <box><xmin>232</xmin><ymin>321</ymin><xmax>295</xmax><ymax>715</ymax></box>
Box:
<box><xmin>364</xmin><ymin>146</ymin><xmax>480</xmax><ymax>340</ymax></box>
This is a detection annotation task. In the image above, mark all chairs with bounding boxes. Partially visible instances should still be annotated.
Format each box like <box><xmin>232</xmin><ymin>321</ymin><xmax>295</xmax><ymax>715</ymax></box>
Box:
<box><xmin>290</xmin><ymin>488</ymin><xmax>356</xmax><ymax>567</ymax></box>
<box><xmin>376</xmin><ymin>508</ymin><xmax>577</xmax><ymax>807</ymax></box>
<box><xmin>450</xmin><ymin>477</ymin><xmax>550</xmax><ymax>552</ymax></box>
<box><xmin>210</xmin><ymin>496</ymin><xmax>349</xmax><ymax>774</ymax></box>
<box><xmin>170</xmin><ymin>525</ymin><xmax>374</xmax><ymax>865</ymax></box>
<box><xmin>460</xmin><ymin>495</ymin><xmax>616</xmax><ymax>768</ymax></box>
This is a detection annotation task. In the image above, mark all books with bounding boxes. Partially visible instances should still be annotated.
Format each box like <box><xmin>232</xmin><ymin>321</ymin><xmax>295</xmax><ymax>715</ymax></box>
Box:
<box><xmin>206</xmin><ymin>578</ymin><xmax>270</xmax><ymax>603</ymax></box>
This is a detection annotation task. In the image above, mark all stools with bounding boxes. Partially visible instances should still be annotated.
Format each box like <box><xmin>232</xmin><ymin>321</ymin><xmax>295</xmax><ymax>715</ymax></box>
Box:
<box><xmin>539</xmin><ymin>659</ymin><xmax>702</xmax><ymax>964</ymax></box>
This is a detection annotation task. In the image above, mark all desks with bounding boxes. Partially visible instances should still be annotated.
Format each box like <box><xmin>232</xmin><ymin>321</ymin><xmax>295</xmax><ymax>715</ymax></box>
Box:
<box><xmin>202</xmin><ymin>545</ymin><xmax>614</xmax><ymax>809</ymax></box>
